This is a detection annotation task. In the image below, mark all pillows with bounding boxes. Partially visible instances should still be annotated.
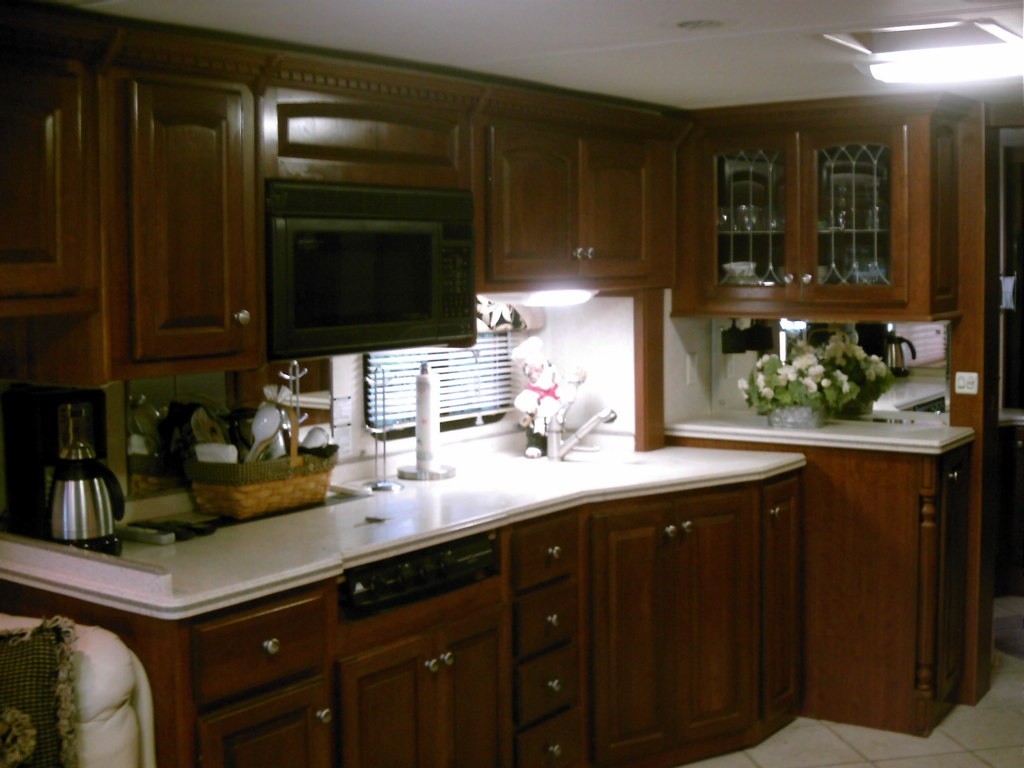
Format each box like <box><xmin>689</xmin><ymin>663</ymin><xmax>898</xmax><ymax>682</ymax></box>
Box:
<box><xmin>0</xmin><ymin>616</ymin><xmax>79</xmax><ymax>768</ymax></box>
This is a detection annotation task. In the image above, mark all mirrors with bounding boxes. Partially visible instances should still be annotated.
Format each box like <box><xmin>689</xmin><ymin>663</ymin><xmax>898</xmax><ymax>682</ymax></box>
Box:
<box><xmin>759</xmin><ymin>320</ymin><xmax>951</xmax><ymax>428</ymax></box>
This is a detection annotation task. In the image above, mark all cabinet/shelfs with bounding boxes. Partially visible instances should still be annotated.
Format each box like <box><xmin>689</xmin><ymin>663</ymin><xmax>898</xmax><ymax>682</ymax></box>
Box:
<box><xmin>0</xmin><ymin>1</ymin><xmax>260</xmax><ymax>382</ymax></box>
<box><xmin>336</xmin><ymin>524</ymin><xmax>513</xmax><ymax>768</ymax></box>
<box><xmin>511</xmin><ymin>505</ymin><xmax>581</xmax><ymax>768</ymax></box>
<box><xmin>590</xmin><ymin>479</ymin><xmax>800</xmax><ymax>768</ymax></box>
<box><xmin>255</xmin><ymin>49</ymin><xmax>488</xmax><ymax>191</ymax></box>
<box><xmin>472</xmin><ymin>80</ymin><xmax>687</xmax><ymax>295</ymax></box>
<box><xmin>669</xmin><ymin>94</ymin><xmax>968</xmax><ymax>321</ymax></box>
<box><xmin>663</xmin><ymin>413</ymin><xmax>975</xmax><ymax>739</ymax></box>
<box><xmin>0</xmin><ymin>574</ymin><xmax>339</xmax><ymax>768</ymax></box>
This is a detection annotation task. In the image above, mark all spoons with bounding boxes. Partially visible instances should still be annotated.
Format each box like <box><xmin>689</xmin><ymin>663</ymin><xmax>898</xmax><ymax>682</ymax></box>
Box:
<box><xmin>244</xmin><ymin>406</ymin><xmax>281</xmax><ymax>461</ymax></box>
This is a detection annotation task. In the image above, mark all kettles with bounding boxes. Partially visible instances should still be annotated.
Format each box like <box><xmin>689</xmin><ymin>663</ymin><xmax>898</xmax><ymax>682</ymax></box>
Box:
<box><xmin>883</xmin><ymin>334</ymin><xmax>916</xmax><ymax>377</ymax></box>
<box><xmin>48</xmin><ymin>440</ymin><xmax>123</xmax><ymax>557</ymax></box>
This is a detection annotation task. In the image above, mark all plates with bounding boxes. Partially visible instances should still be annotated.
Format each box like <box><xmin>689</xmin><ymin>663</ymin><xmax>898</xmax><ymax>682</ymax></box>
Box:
<box><xmin>777</xmin><ymin>266</ymin><xmax>829</xmax><ymax>285</ymax></box>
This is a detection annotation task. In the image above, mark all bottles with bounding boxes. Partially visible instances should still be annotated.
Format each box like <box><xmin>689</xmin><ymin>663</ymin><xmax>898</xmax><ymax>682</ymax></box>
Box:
<box><xmin>835</xmin><ymin>186</ymin><xmax>854</xmax><ymax>229</ymax></box>
<box><xmin>865</xmin><ymin>182</ymin><xmax>889</xmax><ymax>229</ymax></box>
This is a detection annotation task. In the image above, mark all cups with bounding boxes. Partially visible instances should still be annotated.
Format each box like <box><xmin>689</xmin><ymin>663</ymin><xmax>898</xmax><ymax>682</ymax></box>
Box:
<box><xmin>58</xmin><ymin>401</ymin><xmax>93</xmax><ymax>451</ymax></box>
<box><xmin>721</xmin><ymin>261</ymin><xmax>756</xmax><ymax>286</ymax></box>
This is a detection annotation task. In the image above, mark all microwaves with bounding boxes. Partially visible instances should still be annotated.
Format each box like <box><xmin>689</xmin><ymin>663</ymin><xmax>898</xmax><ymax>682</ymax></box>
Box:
<box><xmin>262</xmin><ymin>179</ymin><xmax>476</xmax><ymax>360</ymax></box>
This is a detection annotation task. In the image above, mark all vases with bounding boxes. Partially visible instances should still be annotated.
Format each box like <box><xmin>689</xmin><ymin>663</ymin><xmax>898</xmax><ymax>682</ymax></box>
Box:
<box><xmin>766</xmin><ymin>403</ymin><xmax>827</xmax><ymax>429</ymax></box>
<box><xmin>830</xmin><ymin>398</ymin><xmax>873</xmax><ymax>414</ymax></box>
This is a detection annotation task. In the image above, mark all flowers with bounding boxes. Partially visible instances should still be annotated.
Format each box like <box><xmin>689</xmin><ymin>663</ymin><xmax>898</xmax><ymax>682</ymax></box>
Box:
<box><xmin>814</xmin><ymin>336</ymin><xmax>896</xmax><ymax>402</ymax></box>
<box><xmin>738</xmin><ymin>342</ymin><xmax>861</xmax><ymax>416</ymax></box>
